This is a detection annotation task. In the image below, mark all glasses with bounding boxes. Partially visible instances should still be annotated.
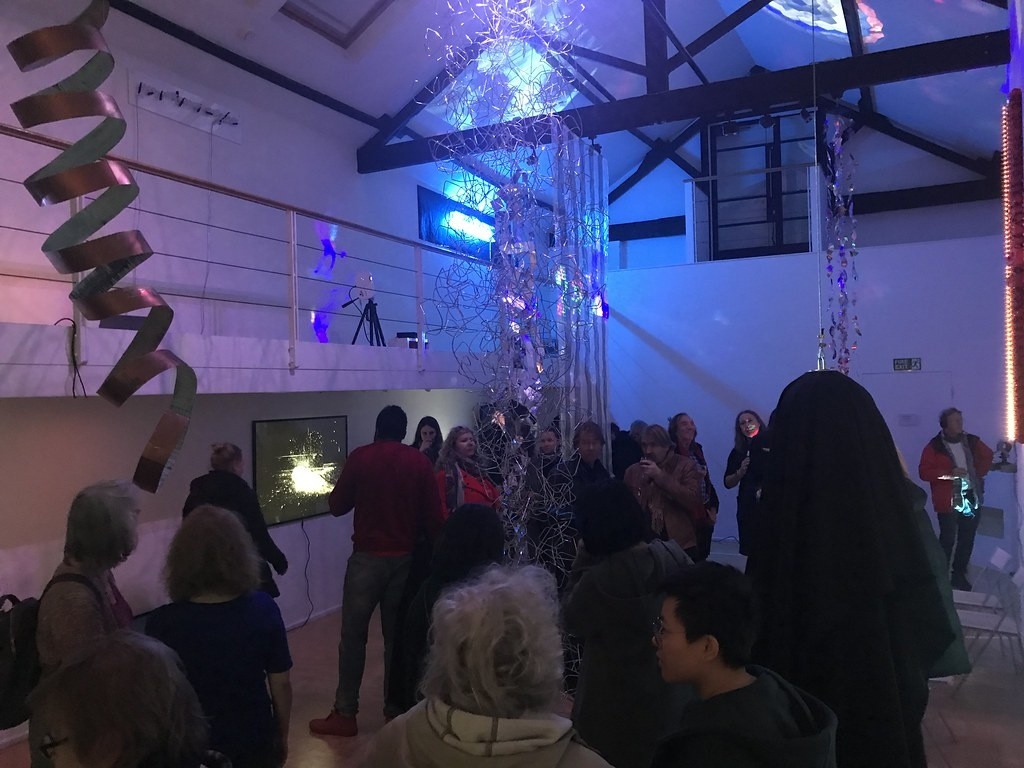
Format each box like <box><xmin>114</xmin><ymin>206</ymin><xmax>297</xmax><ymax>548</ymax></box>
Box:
<box><xmin>652</xmin><ymin>616</ymin><xmax>685</xmax><ymax>648</ymax></box>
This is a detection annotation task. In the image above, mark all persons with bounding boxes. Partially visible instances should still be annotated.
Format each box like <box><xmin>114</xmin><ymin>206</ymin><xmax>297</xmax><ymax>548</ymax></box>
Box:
<box><xmin>29</xmin><ymin>444</ymin><xmax>293</xmax><ymax>768</ymax></box>
<box><xmin>309</xmin><ymin>371</ymin><xmax>971</xmax><ymax>768</ymax></box>
<box><xmin>919</xmin><ymin>408</ymin><xmax>994</xmax><ymax>592</ymax></box>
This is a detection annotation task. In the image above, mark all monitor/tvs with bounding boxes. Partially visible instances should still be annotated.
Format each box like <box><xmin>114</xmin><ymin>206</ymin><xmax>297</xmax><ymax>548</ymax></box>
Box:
<box><xmin>252</xmin><ymin>415</ymin><xmax>347</xmax><ymax>527</ymax></box>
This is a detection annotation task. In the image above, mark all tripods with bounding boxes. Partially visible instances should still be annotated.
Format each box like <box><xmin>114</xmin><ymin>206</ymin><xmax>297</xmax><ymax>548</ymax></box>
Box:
<box><xmin>342</xmin><ymin>297</ymin><xmax>387</xmax><ymax>348</ymax></box>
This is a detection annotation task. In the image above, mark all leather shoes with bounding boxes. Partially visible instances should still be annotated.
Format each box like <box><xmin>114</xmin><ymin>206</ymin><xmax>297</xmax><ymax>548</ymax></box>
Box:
<box><xmin>383</xmin><ymin>702</ymin><xmax>419</xmax><ymax>718</ymax></box>
<box><xmin>950</xmin><ymin>573</ymin><xmax>970</xmax><ymax>592</ymax></box>
<box><xmin>309</xmin><ymin>711</ymin><xmax>358</xmax><ymax>736</ymax></box>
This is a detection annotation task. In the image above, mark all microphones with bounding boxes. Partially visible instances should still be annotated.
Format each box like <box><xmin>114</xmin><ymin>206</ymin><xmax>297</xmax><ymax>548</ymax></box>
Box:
<box><xmin>745</xmin><ymin>437</ymin><xmax>752</xmax><ymax>458</ymax></box>
